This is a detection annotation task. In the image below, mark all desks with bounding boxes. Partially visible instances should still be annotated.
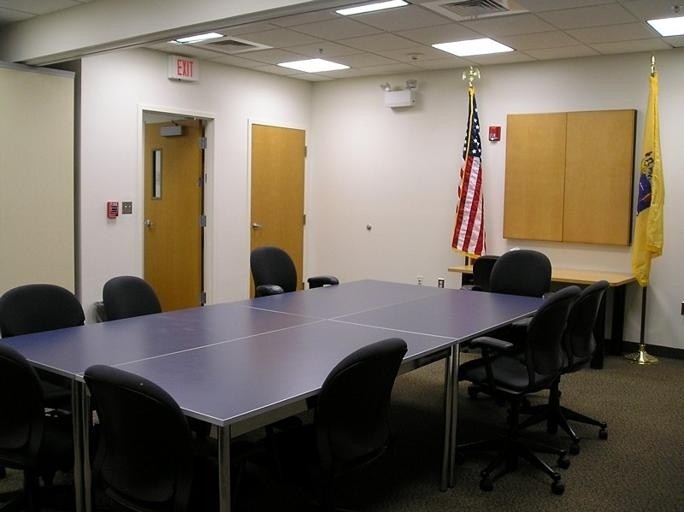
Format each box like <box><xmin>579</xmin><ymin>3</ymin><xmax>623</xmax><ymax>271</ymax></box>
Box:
<box><xmin>447</xmin><ymin>265</ymin><xmax>637</xmax><ymax>356</ymax></box>
<box><xmin>1</xmin><ymin>279</ymin><xmax>548</xmax><ymax>512</ymax></box>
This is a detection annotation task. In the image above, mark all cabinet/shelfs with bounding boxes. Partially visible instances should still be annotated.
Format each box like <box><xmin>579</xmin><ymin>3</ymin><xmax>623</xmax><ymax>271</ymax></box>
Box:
<box><xmin>503</xmin><ymin>108</ymin><xmax>637</xmax><ymax>247</ymax></box>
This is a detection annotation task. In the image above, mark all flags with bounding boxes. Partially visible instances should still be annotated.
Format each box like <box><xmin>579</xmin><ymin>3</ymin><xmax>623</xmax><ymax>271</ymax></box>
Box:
<box><xmin>632</xmin><ymin>75</ymin><xmax>665</xmax><ymax>287</ymax></box>
<box><xmin>451</xmin><ymin>88</ymin><xmax>487</xmax><ymax>265</ymax></box>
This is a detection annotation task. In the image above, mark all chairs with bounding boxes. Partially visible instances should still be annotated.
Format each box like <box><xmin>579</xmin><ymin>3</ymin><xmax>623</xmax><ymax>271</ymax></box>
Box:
<box><xmin>103</xmin><ymin>276</ymin><xmax>162</xmax><ymax>321</ymax></box>
<box><xmin>0</xmin><ymin>284</ymin><xmax>96</xmax><ymax>416</ymax></box>
<box><xmin>458</xmin><ymin>285</ymin><xmax>583</xmax><ymax>495</ymax></box>
<box><xmin>468</xmin><ymin>249</ymin><xmax>562</xmax><ymax>406</ymax></box>
<box><xmin>211</xmin><ymin>338</ymin><xmax>407</xmax><ymax>511</ymax></box>
<box><xmin>470</xmin><ymin>255</ymin><xmax>500</xmax><ymax>291</ymax></box>
<box><xmin>83</xmin><ymin>365</ymin><xmax>215</xmax><ymax>512</ymax></box>
<box><xmin>0</xmin><ymin>342</ymin><xmax>73</xmax><ymax>512</ymax></box>
<box><xmin>506</xmin><ymin>280</ymin><xmax>609</xmax><ymax>456</ymax></box>
<box><xmin>250</xmin><ymin>247</ymin><xmax>339</xmax><ymax>298</ymax></box>
